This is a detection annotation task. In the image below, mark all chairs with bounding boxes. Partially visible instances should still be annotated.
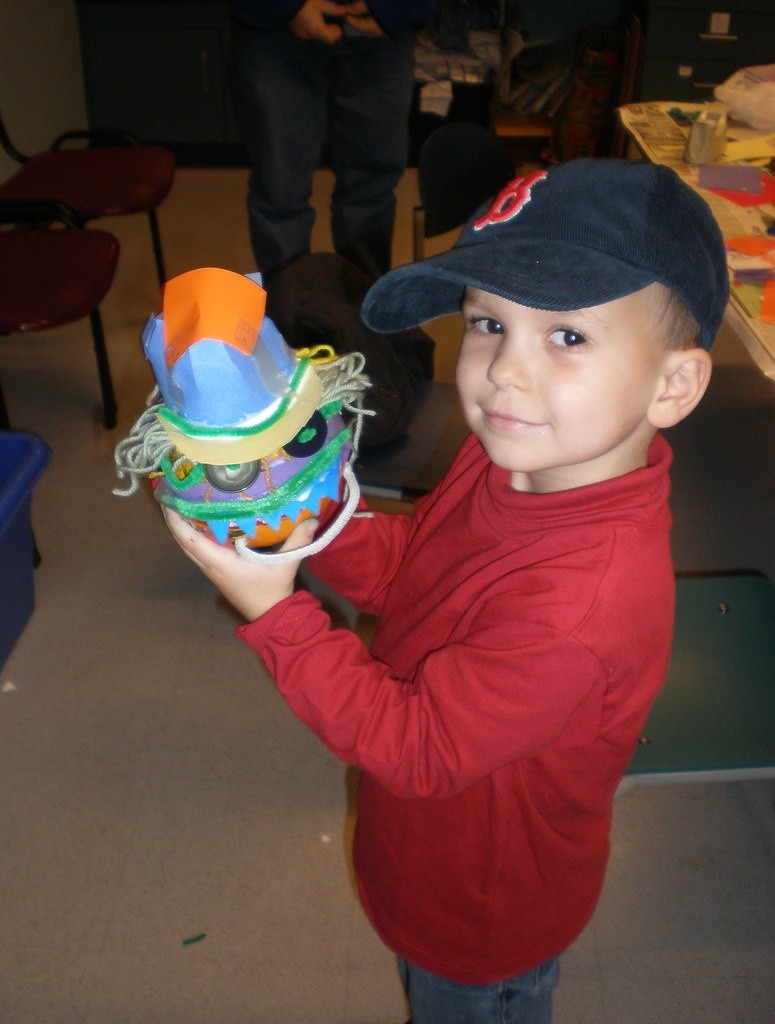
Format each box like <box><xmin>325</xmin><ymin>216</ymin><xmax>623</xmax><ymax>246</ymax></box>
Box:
<box><xmin>358</xmin><ymin>382</ymin><xmax>471</xmax><ymax>502</ymax></box>
<box><xmin>615</xmin><ymin>576</ymin><xmax>775</xmax><ymax>796</ymax></box>
<box><xmin>491</xmin><ymin>113</ymin><xmax>556</xmax><ymax>137</ymax></box>
<box><xmin>0</xmin><ymin>197</ymin><xmax>120</xmax><ymax>431</ymax></box>
<box><xmin>1</xmin><ymin>116</ymin><xmax>176</xmax><ymax>290</ymax></box>
<box><xmin>418</xmin><ymin>123</ymin><xmax>516</xmax><ymax>236</ymax></box>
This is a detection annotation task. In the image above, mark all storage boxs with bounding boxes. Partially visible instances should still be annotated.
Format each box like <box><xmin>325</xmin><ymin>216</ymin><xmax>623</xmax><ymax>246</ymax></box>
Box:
<box><xmin>0</xmin><ymin>429</ymin><xmax>54</xmax><ymax>675</ymax></box>
<box><xmin>406</xmin><ymin>80</ymin><xmax>495</xmax><ymax>167</ymax></box>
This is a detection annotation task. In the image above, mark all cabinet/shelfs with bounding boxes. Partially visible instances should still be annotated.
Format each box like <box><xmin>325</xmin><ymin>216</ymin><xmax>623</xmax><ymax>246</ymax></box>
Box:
<box><xmin>640</xmin><ymin>0</ymin><xmax>775</xmax><ymax>98</ymax></box>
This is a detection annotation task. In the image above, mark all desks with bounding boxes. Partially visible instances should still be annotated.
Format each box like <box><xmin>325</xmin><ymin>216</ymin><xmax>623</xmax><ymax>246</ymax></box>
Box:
<box><xmin>616</xmin><ymin>100</ymin><xmax>775</xmax><ymax>380</ymax></box>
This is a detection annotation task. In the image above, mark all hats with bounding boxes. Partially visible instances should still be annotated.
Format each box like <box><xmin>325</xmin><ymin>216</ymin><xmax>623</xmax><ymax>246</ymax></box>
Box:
<box><xmin>361</xmin><ymin>158</ymin><xmax>730</xmax><ymax>350</ymax></box>
<box><xmin>418</xmin><ymin>123</ymin><xmax>514</xmax><ymax>238</ymax></box>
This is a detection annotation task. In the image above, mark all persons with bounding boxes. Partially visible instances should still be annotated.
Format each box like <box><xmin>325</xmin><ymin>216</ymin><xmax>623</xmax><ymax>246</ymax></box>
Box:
<box><xmin>159</xmin><ymin>156</ymin><xmax>729</xmax><ymax>1023</ymax></box>
<box><xmin>233</xmin><ymin>0</ymin><xmax>416</xmax><ymax>295</ymax></box>
